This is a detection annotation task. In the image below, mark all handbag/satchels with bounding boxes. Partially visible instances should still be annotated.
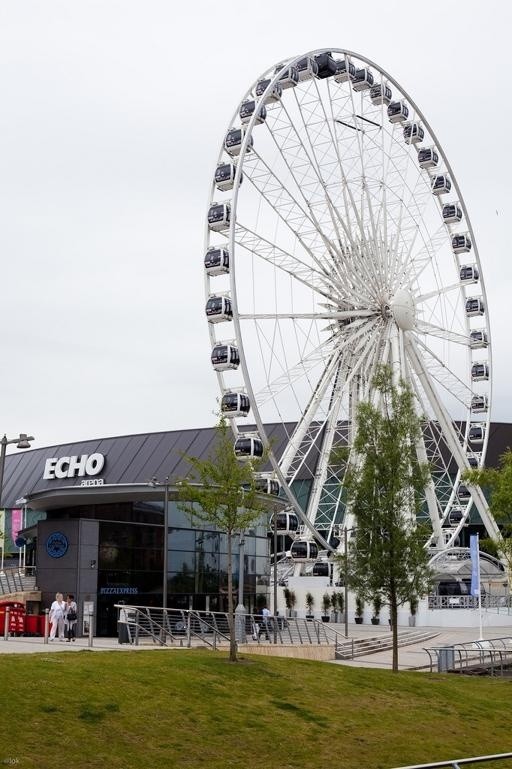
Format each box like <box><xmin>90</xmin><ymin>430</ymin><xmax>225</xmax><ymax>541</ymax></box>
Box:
<box><xmin>66</xmin><ymin>614</ymin><xmax>76</xmax><ymax>620</ymax></box>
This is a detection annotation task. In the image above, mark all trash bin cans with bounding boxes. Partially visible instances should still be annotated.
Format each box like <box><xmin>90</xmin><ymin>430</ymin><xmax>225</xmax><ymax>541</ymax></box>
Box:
<box><xmin>117</xmin><ymin>609</ymin><xmax>136</xmax><ymax>644</ymax></box>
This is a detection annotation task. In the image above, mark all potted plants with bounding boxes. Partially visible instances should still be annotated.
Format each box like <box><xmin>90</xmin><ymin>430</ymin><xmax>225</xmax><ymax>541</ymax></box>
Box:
<box><xmin>321</xmin><ymin>590</ymin><xmax>331</xmax><ymax>622</ymax></box>
<box><xmin>283</xmin><ymin>589</ymin><xmax>297</xmax><ymax>620</ymax></box>
<box><xmin>331</xmin><ymin>591</ymin><xmax>337</xmax><ymax>623</ymax></box>
<box><xmin>409</xmin><ymin>595</ymin><xmax>419</xmax><ymax>626</ymax></box>
<box><xmin>304</xmin><ymin>593</ymin><xmax>314</xmax><ymax>622</ymax></box>
<box><xmin>337</xmin><ymin>592</ymin><xmax>344</xmax><ymax>623</ymax></box>
<box><xmin>355</xmin><ymin>595</ymin><xmax>363</xmax><ymax>624</ymax></box>
<box><xmin>371</xmin><ymin>596</ymin><xmax>381</xmax><ymax>625</ymax></box>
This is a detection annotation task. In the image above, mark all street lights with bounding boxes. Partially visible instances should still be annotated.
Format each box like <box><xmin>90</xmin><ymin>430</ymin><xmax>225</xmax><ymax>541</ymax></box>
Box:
<box><xmin>1</xmin><ymin>432</ymin><xmax>36</xmax><ymax>503</ymax></box>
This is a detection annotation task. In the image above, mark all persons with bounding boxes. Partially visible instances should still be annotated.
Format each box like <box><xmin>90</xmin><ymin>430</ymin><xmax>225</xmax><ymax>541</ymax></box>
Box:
<box><xmin>63</xmin><ymin>595</ymin><xmax>78</xmax><ymax>642</ymax></box>
<box><xmin>48</xmin><ymin>592</ymin><xmax>66</xmax><ymax>642</ymax></box>
<box><xmin>259</xmin><ymin>605</ymin><xmax>271</xmax><ymax>640</ymax></box>
<box><xmin>251</xmin><ymin>608</ymin><xmax>260</xmax><ymax>640</ymax></box>
